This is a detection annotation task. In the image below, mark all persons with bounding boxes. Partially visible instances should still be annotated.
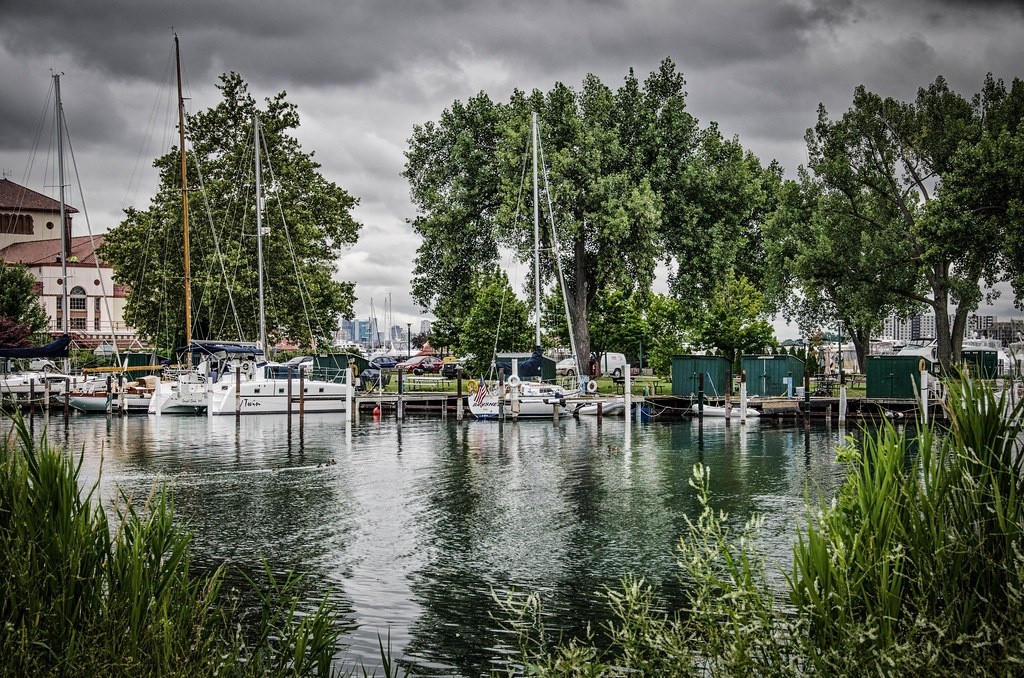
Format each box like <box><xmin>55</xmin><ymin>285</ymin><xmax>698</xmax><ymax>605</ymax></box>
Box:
<box><xmin>56</xmin><ymin>253</ymin><xmax>62</xmax><ymax>263</ymax></box>
<box><xmin>70</xmin><ymin>252</ymin><xmax>79</xmax><ymax>263</ymax></box>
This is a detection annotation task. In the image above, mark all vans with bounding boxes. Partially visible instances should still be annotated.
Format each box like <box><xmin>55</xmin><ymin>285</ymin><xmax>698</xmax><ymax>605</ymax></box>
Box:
<box><xmin>589</xmin><ymin>351</ymin><xmax>627</xmax><ymax>377</ymax></box>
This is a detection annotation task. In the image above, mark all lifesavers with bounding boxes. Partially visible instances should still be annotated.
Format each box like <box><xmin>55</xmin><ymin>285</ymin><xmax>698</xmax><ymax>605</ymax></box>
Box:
<box><xmin>508</xmin><ymin>374</ymin><xmax>521</xmax><ymax>388</ymax></box>
<box><xmin>467</xmin><ymin>380</ymin><xmax>479</xmax><ymax>393</ymax></box>
<box><xmin>919</xmin><ymin>359</ymin><xmax>925</xmax><ymax>372</ymax></box>
<box><xmin>348</xmin><ymin>364</ymin><xmax>359</xmax><ymax>376</ymax></box>
<box><xmin>586</xmin><ymin>380</ymin><xmax>597</xmax><ymax>393</ymax></box>
<box><xmin>82</xmin><ymin>381</ymin><xmax>95</xmax><ymax>395</ymax></box>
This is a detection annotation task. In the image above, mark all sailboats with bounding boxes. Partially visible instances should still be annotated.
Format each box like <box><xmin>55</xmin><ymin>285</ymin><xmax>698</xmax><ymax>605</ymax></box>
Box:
<box><xmin>50</xmin><ymin>24</ymin><xmax>247</xmax><ymax>414</ymax></box>
<box><xmin>466</xmin><ymin>113</ymin><xmax>584</xmax><ymax>421</ymax></box>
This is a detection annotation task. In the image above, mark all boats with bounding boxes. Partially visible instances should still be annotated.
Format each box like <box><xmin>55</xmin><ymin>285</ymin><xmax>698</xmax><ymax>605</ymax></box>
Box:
<box><xmin>690</xmin><ymin>403</ymin><xmax>761</xmax><ymax>418</ymax></box>
<box><xmin>148</xmin><ymin>108</ymin><xmax>354</xmax><ymax>416</ymax></box>
<box><xmin>895</xmin><ymin>334</ymin><xmax>1024</xmax><ymax>428</ymax></box>
<box><xmin>0</xmin><ymin>67</ymin><xmax>124</xmax><ymax>410</ymax></box>
<box><xmin>575</xmin><ymin>397</ymin><xmax>625</xmax><ymax>416</ymax></box>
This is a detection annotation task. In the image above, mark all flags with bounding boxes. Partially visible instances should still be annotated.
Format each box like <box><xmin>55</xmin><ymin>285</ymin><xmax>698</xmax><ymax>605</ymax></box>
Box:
<box><xmin>474</xmin><ymin>377</ymin><xmax>488</xmax><ymax>405</ymax></box>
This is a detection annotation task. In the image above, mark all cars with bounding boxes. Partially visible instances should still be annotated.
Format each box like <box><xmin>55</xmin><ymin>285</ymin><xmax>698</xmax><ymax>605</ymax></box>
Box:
<box><xmin>18</xmin><ymin>357</ymin><xmax>56</xmax><ymax>373</ymax></box>
<box><xmin>371</xmin><ymin>356</ymin><xmax>397</xmax><ymax>368</ymax></box>
<box><xmin>281</xmin><ymin>354</ymin><xmax>313</xmax><ymax>374</ymax></box>
<box><xmin>394</xmin><ymin>354</ymin><xmax>444</xmax><ymax>373</ymax></box>
<box><xmin>555</xmin><ymin>358</ymin><xmax>577</xmax><ymax>376</ymax></box>
<box><xmin>442</xmin><ymin>361</ymin><xmax>462</xmax><ymax>379</ymax></box>
